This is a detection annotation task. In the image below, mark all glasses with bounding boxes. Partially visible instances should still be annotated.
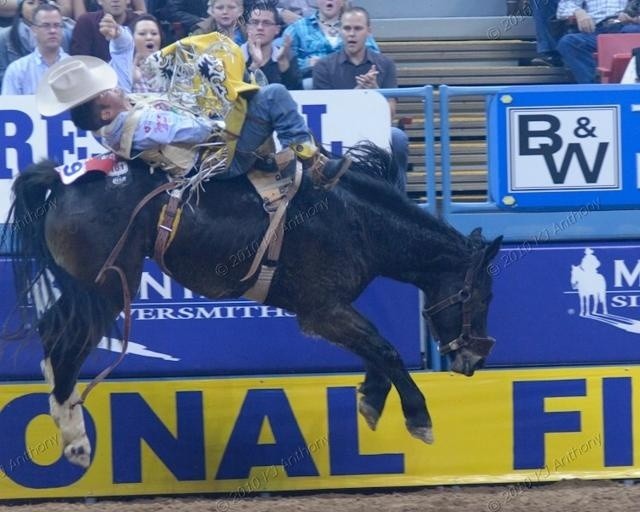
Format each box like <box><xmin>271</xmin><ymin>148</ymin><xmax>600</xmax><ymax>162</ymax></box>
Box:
<box><xmin>35</xmin><ymin>23</ymin><xmax>64</xmax><ymax>29</ymax></box>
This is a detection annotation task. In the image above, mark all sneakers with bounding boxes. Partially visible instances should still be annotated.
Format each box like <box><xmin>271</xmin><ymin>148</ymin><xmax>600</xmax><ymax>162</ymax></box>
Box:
<box><xmin>532</xmin><ymin>52</ymin><xmax>562</xmax><ymax>68</ymax></box>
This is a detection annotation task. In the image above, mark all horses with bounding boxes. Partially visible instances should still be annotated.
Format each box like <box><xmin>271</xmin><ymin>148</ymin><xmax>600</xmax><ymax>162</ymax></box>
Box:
<box><xmin>0</xmin><ymin>139</ymin><xmax>504</xmax><ymax>468</ymax></box>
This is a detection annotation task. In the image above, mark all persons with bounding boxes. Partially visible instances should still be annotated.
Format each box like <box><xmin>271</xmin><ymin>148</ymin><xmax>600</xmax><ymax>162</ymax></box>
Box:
<box><xmin>505</xmin><ymin>0</ymin><xmax>640</xmax><ymax>84</ymax></box>
<box><xmin>0</xmin><ymin>0</ymin><xmax>411</xmax><ymax>198</ymax></box>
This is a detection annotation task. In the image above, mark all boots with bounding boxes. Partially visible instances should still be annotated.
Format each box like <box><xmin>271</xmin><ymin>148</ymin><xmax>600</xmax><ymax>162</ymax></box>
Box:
<box><xmin>300</xmin><ymin>153</ymin><xmax>350</xmax><ymax>192</ymax></box>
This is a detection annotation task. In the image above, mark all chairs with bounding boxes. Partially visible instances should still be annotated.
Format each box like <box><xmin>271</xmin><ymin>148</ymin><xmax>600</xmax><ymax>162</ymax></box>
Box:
<box><xmin>596</xmin><ymin>34</ymin><xmax>639</xmax><ymax>86</ymax></box>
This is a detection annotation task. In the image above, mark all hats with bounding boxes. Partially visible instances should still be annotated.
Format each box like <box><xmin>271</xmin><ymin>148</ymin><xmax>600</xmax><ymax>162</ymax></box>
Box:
<box><xmin>35</xmin><ymin>54</ymin><xmax>119</xmax><ymax>117</ymax></box>
